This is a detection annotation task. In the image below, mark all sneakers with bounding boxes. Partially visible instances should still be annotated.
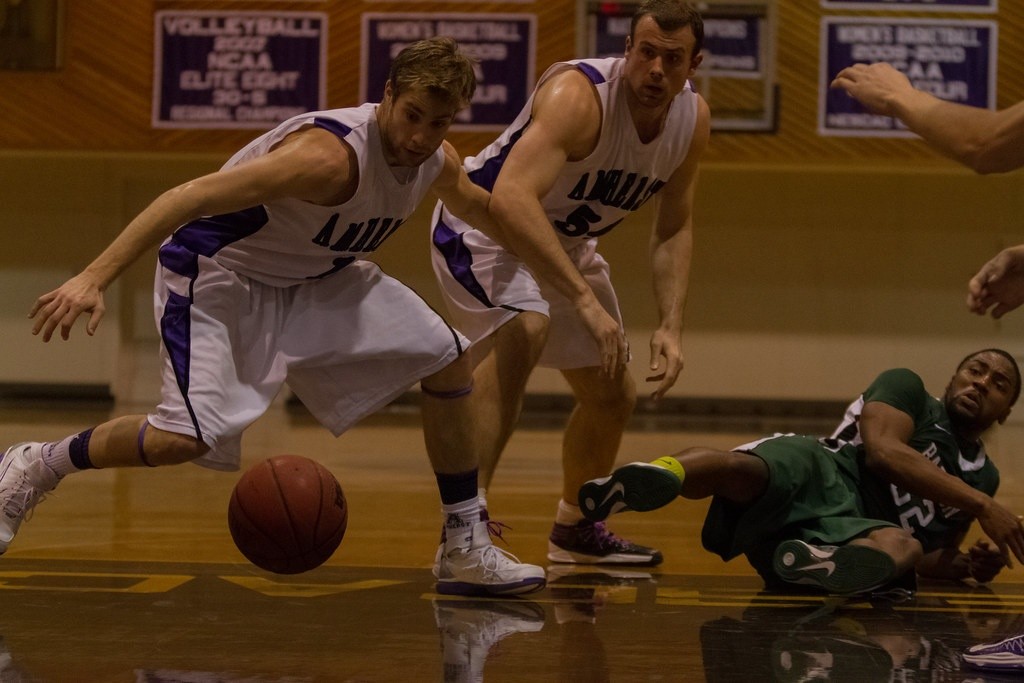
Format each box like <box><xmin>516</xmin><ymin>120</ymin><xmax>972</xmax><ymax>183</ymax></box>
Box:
<box><xmin>435</xmin><ymin>522</ymin><xmax>547</xmax><ymax>597</ymax></box>
<box><xmin>432</xmin><ymin>599</ymin><xmax>546</xmax><ymax>672</ymax></box>
<box><xmin>962</xmin><ymin>634</ymin><xmax>1024</xmax><ymax>671</ymax></box>
<box><xmin>0</xmin><ymin>441</ymin><xmax>60</xmax><ymax>553</ymax></box>
<box><xmin>547</xmin><ymin>519</ymin><xmax>663</xmax><ymax>566</ymax></box>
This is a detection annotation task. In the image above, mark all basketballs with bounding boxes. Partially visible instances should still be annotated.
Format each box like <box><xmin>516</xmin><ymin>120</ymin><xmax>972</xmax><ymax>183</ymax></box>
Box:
<box><xmin>227</xmin><ymin>453</ymin><xmax>350</xmax><ymax>576</ymax></box>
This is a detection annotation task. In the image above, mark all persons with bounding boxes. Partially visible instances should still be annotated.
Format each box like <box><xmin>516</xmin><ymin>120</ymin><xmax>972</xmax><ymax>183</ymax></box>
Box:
<box><xmin>432</xmin><ymin>0</ymin><xmax>713</xmax><ymax>566</ymax></box>
<box><xmin>578</xmin><ymin>349</ymin><xmax>1023</xmax><ymax>604</ymax></box>
<box><xmin>696</xmin><ymin>601</ymin><xmax>1024</xmax><ymax>683</ymax></box>
<box><xmin>0</xmin><ymin>38</ymin><xmax>546</xmax><ymax>598</ymax></box>
<box><xmin>549</xmin><ymin>565</ymin><xmax>662</xmax><ymax>683</ymax></box>
<box><xmin>430</xmin><ymin>599</ymin><xmax>545</xmax><ymax>683</ymax></box>
<box><xmin>830</xmin><ymin>63</ymin><xmax>1024</xmax><ymax>320</ymax></box>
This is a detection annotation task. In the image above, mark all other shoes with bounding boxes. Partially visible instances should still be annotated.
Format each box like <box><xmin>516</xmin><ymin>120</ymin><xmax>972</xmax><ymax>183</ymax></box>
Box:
<box><xmin>771</xmin><ymin>538</ymin><xmax>896</xmax><ymax>598</ymax></box>
<box><xmin>769</xmin><ymin>636</ymin><xmax>893</xmax><ymax>683</ymax></box>
<box><xmin>577</xmin><ymin>462</ymin><xmax>682</xmax><ymax>522</ymax></box>
